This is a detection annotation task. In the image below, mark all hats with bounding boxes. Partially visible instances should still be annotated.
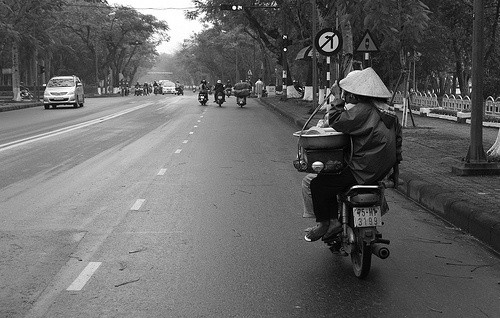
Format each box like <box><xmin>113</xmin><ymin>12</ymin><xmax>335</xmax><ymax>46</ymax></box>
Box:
<box><xmin>339</xmin><ymin>66</ymin><xmax>393</xmax><ymax>98</ymax></box>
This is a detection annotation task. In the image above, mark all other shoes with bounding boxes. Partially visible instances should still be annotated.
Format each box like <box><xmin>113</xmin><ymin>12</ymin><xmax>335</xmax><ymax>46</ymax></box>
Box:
<box><xmin>304</xmin><ymin>224</ymin><xmax>320</xmax><ymax>232</ymax></box>
<box><xmin>321</xmin><ymin>223</ymin><xmax>343</xmax><ymax>241</ymax></box>
<box><xmin>304</xmin><ymin>222</ymin><xmax>329</xmax><ymax>242</ymax></box>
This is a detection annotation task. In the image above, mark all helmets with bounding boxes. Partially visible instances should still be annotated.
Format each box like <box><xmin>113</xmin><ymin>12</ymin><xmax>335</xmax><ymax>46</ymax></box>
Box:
<box><xmin>217</xmin><ymin>80</ymin><xmax>222</xmax><ymax>83</ymax></box>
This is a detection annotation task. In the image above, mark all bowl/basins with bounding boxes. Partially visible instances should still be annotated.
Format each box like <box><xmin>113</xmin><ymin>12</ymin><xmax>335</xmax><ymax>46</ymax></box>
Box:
<box><xmin>293</xmin><ymin>128</ymin><xmax>345</xmax><ymax>150</ymax></box>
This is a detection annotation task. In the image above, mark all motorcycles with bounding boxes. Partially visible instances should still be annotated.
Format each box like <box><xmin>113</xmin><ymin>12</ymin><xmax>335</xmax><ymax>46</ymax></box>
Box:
<box><xmin>324</xmin><ymin>184</ymin><xmax>390</xmax><ymax>278</ymax></box>
<box><xmin>198</xmin><ymin>90</ymin><xmax>210</xmax><ymax>106</ymax></box>
<box><xmin>213</xmin><ymin>85</ymin><xmax>226</xmax><ymax>106</ymax></box>
<box><xmin>226</xmin><ymin>89</ymin><xmax>232</xmax><ymax>98</ymax></box>
<box><xmin>237</xmin><ymin>95</ymin><xmax>246</xmax><ymax>108</ymax></box>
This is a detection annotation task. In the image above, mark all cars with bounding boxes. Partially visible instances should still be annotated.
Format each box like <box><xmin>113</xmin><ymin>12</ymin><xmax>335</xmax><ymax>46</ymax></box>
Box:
<box><xmin>42</xmin><ymin>75</ymin><xmax>85</xmax><ymax>110</ymax></box>
<box><xmin>161</xmin><ymin>82</ymin><xmax>177</xmax><ymax>95</ymax></box>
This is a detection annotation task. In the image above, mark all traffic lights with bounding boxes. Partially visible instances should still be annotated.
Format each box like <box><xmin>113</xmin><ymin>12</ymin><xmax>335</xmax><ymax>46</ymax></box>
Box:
<box><xmin>282</xmin><ymin>34</ymin><xmax>287</xmax><ymax>52</ymax></box>
<box><xmin>220</xmin><ymin>3</ymin><xmax>243</xmax><ymax>11</ymax></box>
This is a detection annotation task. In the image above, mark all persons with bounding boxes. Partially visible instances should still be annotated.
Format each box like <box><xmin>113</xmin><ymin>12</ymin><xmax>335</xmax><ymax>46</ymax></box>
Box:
<box><xmin>301</xmin><ymin>67</ymin><xmax>402</xmax><ymax>241</ymax></box>
<box><xmin>20</xmin><ymin>82</ymin><xmax>29</xmax><ymax>96</ymax></box>
<box><xmin>119</xmin><ymin>81</ymin><xmax>183</xmax><ymax>96</ymax></box>
<box><xmin>193</xmin><ymin>79</ymin><xmax>263</xmax><ymax>106</ymax></box>
